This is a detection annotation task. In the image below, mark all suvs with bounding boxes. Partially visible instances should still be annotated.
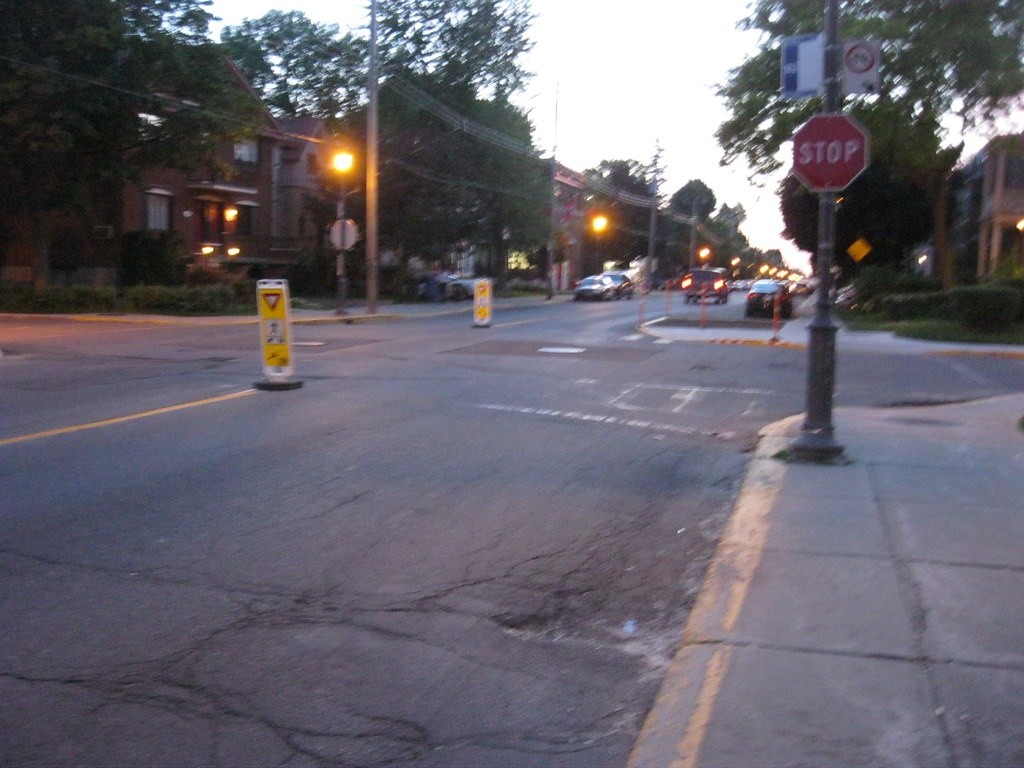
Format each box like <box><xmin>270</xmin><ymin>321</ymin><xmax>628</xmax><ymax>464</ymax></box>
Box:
<box><xmin>683</xmin><ymin>267</ymin><xmax>728</xmax><ymax>305</ymax></box>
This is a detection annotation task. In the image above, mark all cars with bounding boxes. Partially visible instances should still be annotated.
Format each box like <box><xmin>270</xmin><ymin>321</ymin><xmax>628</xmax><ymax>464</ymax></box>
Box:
<box><xmin>744</xmin><ymin>279</ymin><xmax>793</xmax><ymax>318</ymax></box>
<box><xmin>599</xmin><ymin>270</ymin><xmax>636</xmax><ymax>300</ymax></box>
<box><xmin>725</xmin><ymin>277</ymin><xmax>811</xmax><ymax>296</ymax></box>
<box><xmin>417</xmin><ymin>273</ymin><xmax>496</xmax><ymax>302</ymax></box>
<box><xmin>573</xmin><ymin>275</ymin><xmax>617</xmax><ymax>302</ymax></box>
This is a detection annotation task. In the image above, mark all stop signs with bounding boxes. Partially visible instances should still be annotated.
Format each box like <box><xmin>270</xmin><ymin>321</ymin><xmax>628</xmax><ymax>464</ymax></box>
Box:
<box><xmin>791</xmin><ymin>113</ymin><xmax>868</xmax><ymax>193</ymax></box>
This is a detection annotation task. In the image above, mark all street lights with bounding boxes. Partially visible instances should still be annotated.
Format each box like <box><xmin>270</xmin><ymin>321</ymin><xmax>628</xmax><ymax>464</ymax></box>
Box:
<box><xmin>329</xmin><ymin>152</ymin><xmax>351</xmax><ymax>314</ymax></box>
